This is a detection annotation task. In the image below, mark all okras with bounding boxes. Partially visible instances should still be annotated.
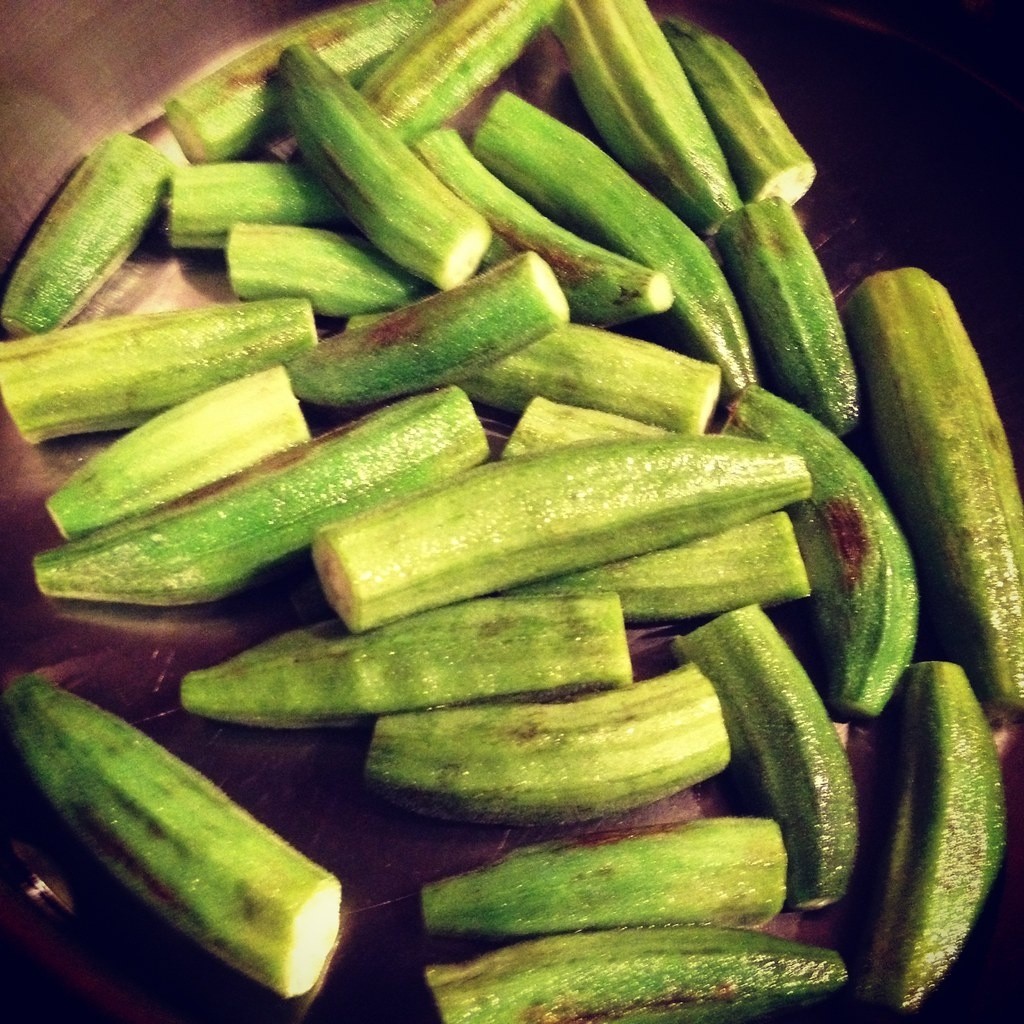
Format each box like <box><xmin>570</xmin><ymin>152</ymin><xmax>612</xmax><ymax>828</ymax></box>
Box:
<box><xmin>0</xmin><ymin>0</ymin><xmax>1024</xmax><ymax>1024</ymax></box>
